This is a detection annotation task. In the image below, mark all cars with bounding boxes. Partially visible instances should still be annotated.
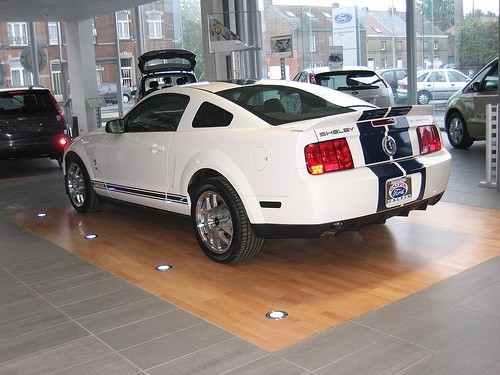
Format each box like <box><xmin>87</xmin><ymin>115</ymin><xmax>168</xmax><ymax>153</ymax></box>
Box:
<box><xmin>376</xmin><ymin>68</ymin><xmax>407</xmax><ymax>91</ymax></box>
<box><xmin>444</xmin><ymin>57</ymin><xmax>499</xmax><ymax>149</ymax></box>
<box><xmin>135</xmin><ymin>48</ymin><xmax>198</xmax><ymax>105</ymax></box>
<box><xmin>98</xmin><ymin>84</ymin><xmax>131</xmax><ymax>104</ymax></box>
<box><xmin>292</xmin><ymin>65</ymin><xmax>395</xmax><ymax>108</ymax></box>
<box><xmin>63</xmin><ymin>77</ymin><xmax>452</xmax><ymax>265</ymax></box>
<box><xmin>396</xmin><ymin>68</ymin><xmax>472</xmax><ymax>105</ymax></box>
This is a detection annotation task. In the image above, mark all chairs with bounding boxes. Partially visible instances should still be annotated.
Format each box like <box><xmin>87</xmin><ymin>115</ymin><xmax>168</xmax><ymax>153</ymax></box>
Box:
<box><xmin>346</xmin><ymin>75</ymin><xmax>359</xmax><ymax>87</ymax></box>
<box><xmin>263</xmin><ymin>98</ymin><xmax>287</xmax><ymax>114</ymax></box>
<box><xmin>147</xmin><ymin>78</ymin><xmax>187</xmax><ymax>95</ymax></box>
<box><xmin>21</xmin><ymin>96</ymin><xmax>36</xmax><ymax>113</ymax></box>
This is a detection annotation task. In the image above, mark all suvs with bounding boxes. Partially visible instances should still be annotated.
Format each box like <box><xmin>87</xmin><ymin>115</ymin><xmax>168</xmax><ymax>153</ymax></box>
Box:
<box><xmin>0</xmin><ymin>84</ymin><xmax>70</xmax><ymax>168</ymax></box>
